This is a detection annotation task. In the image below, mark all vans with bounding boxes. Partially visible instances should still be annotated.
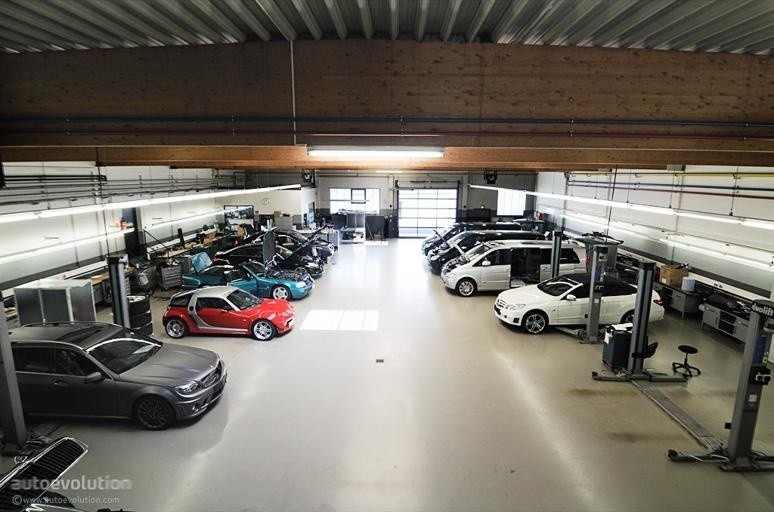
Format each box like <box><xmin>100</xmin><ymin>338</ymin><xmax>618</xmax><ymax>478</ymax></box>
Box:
<box><xmin>440</xmin><ymin>238</ymin><xmax>586</xmax><ymax>297</ymax></box>
<box><xmin>426</xmin><ymin>228</ymin><xmax>545</xmax><ymax>274</ymax></box>
<box><xmin>421</xmin><ymin>221</ymin><xmax>521</xmax><ymax>257</ymax></box>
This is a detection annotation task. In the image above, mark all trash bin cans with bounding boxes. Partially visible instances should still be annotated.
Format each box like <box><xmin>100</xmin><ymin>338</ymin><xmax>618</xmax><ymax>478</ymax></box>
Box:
<box><xmin>127</xmin><ymin>295</ymin><xmax>154</xmax><ymax>337</ymax></box>
<box><xmin>603</xmin><ymin>325</ymin><xmax>633</xmax><ymax>372</ymax></box>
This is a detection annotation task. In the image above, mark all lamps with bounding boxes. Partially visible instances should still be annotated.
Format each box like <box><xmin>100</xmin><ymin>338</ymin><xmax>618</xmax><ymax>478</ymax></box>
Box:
<box><xmin>0</xmin><ymin>183</ymin><xmax>302</xmax><ymax>224</ymax></box>
<box><xmin>469</xmin><ymin>168</ymin><xmax>774</xmax><ymax>231</ymax></box>
<box><xmin>307</xmin><ymin>146</ymin><xmax>444</xmax><ymax>160</ymax></box>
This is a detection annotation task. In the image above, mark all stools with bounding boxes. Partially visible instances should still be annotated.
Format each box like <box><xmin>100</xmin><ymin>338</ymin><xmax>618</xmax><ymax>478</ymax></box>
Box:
<box><xmin>673</xmin><ymin>346</ymin><xmax>701</xmax><ymax>377</ymax></box>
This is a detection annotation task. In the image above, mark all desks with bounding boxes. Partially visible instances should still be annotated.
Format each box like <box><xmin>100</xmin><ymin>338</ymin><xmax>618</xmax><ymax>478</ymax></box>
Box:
<box><xmin>654</xmin><ymin>282</ymin><xmax>706</xmax><ymax>319</ymax></box>
<box><xmin>701</xmin><ymin>299</ymin><xmax>749</xmax><ymax>342</ymax></box>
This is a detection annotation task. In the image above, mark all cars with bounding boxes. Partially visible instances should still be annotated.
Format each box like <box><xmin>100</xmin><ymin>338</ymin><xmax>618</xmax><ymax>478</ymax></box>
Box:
<box><xmin>493</xmin><ymin>272</ymin><xmax>665</xmax><ymax>334</ymax></box>
<box><xmin>162</xmin><ymin>284</ymin><xmax>295</xmax><ymax>340</ymax></box>
<box><xmin>1</xmin><ymin>320</ymin><xmax>227</xmax><ymax>431</ymax></box>
<box><xmin>181</xmin><ymin>229</ymin><xmax>336</xmax><ymax>300</ymax></box>
